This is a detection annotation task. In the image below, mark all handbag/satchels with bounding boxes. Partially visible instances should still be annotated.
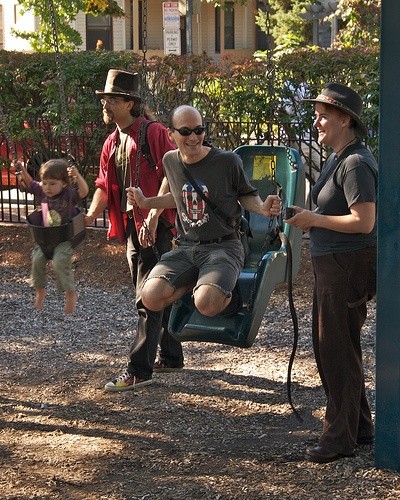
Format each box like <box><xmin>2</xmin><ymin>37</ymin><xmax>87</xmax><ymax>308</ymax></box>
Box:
<box><xmin>241</xmin><ymin>216</ymin><xmax>253</xmax><ymax>256</ymax></box>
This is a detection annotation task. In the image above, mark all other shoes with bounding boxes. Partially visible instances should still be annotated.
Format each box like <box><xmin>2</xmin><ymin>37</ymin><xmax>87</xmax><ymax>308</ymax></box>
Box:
<box><xmin>220</xmin><ymin>281</ymin><xmax>243</xmax><ymax>317</ymax></box>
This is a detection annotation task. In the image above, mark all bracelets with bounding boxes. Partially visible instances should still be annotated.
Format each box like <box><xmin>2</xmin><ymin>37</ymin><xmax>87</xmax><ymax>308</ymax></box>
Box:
<box><xmin>89</xmin><ymin>216</ymin><xmax>93</xmax><ymax>224</ymax></box>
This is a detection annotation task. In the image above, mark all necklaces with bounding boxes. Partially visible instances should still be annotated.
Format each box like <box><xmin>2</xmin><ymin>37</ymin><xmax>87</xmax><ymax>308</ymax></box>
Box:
<box><xmin>334</xmin><ymin>136</ymin><xmax>360</xmax><ymax>160</ymax></box>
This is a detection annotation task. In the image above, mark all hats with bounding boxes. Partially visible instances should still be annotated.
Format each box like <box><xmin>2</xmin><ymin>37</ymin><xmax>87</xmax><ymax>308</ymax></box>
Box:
<box><xmin>95</xmin><ymin>69</ymin><xmax>144</xmax><ymax>104</ymax></box>
<box><xmin>301</xmin><ymin>83</ymin><xmax>368</xmax><ymax>137</ymax></box>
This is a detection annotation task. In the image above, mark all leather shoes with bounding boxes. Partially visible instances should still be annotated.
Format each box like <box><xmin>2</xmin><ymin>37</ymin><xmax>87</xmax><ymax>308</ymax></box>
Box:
<box><xmin>305</xmin><ymin>444</ymin><xmax>344</xmax><ymax>463</ymax></box>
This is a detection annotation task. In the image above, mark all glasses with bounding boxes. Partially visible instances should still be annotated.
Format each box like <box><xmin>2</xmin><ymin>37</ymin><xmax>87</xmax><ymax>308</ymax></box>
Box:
<box><xmin>174</xmin><ymin>124</ymin><xmax>207</xmax><ymax>136</ymax></box>
<box><xmin>100</xmin><ymin>97</ymin><xmax>124</xmax><ymax>104</ymax></box>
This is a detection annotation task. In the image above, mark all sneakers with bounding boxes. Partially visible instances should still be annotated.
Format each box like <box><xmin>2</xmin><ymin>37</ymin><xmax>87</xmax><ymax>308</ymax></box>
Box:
<box><xmin>106</xmin><ymin>371</ymin><xmax>153</xmax><ymax>391</ymax></box>
<box><xmin>153</xmin><ymin>360</ymin><xmax>183</xmax><ymax>372</ymax></box>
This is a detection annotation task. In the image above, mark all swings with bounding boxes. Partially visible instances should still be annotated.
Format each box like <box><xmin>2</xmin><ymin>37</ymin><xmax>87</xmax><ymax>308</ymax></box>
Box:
<box><xmin>132</xmin><ymin>0</ymin><xmax>308</xmax><ymax>350</ymax></box>
<box><xmin>0</xmin><ymin>0</ymin><xmax>88</xmax><ymax>260</ymax></box>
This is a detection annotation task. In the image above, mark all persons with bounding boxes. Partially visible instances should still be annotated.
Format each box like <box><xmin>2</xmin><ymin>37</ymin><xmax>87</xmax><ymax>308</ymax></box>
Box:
<box><xmin>14</xmin><ymin>160</ymin><xmax>89</xmax><ymax>313</ymax></box>
<box><xmin>126</xmin><ymin>105</ymin><xmax>282</xmax><ymax>317</ymax></box>
<box><xmin>283</xmin><ymin>83</ymin><xmax>378</xmax><ymax>462</ymax></box>
<box><xmin>85</xmin><ymin>69</ymin><xmax>185</xmax><ymax>392</ymax></box>
